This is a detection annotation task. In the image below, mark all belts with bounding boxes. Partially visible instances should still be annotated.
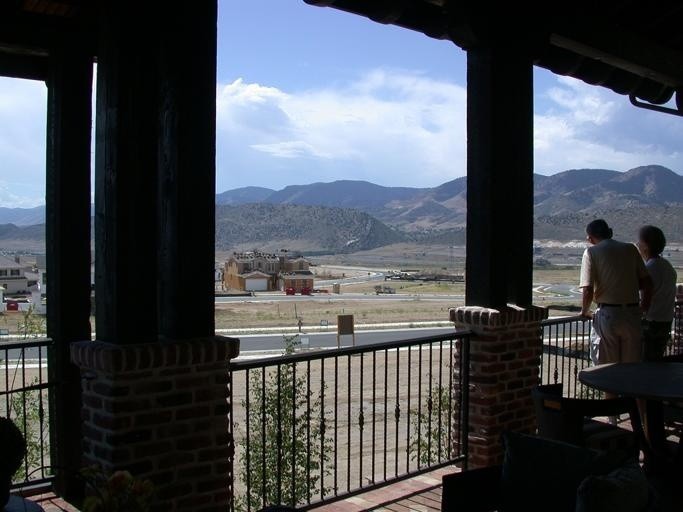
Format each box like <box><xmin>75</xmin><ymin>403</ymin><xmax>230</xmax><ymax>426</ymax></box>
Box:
<box><xmin>598</xmin><ymin>302</ymin><xmax>639</xmax><ymax>308</ymax></box>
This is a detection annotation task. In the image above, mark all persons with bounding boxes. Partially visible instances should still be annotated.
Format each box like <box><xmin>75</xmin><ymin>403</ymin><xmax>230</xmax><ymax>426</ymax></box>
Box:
<box><xmin>631</xmin><ymin>226</ymin><xmax>676</xmax><ymax>359</ymax></box>
<box><xmin>578</xmin><ymin>220</ymin><xmax>656</xmax><ymax>432</ymax></box>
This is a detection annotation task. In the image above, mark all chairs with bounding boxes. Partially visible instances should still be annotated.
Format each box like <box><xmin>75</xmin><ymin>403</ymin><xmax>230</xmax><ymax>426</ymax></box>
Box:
<box><xmin>442</xmin><ymin>385</ymin><xmax>682</xmax><ymax>511</ymax></box>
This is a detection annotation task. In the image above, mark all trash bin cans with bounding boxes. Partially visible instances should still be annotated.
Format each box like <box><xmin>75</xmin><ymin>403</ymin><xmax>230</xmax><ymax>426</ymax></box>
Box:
<box><xmin>333</xmin><ymin>283</ymin><xmax>340</xmax><ymax>294</ymax></box>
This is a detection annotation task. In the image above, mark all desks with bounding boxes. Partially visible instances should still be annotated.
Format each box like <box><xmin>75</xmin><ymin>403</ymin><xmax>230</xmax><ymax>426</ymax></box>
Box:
<box><xmin>579</xmin><ymin>360</ymin><xmax>683</xmax><ymax>472</ymax></box>
